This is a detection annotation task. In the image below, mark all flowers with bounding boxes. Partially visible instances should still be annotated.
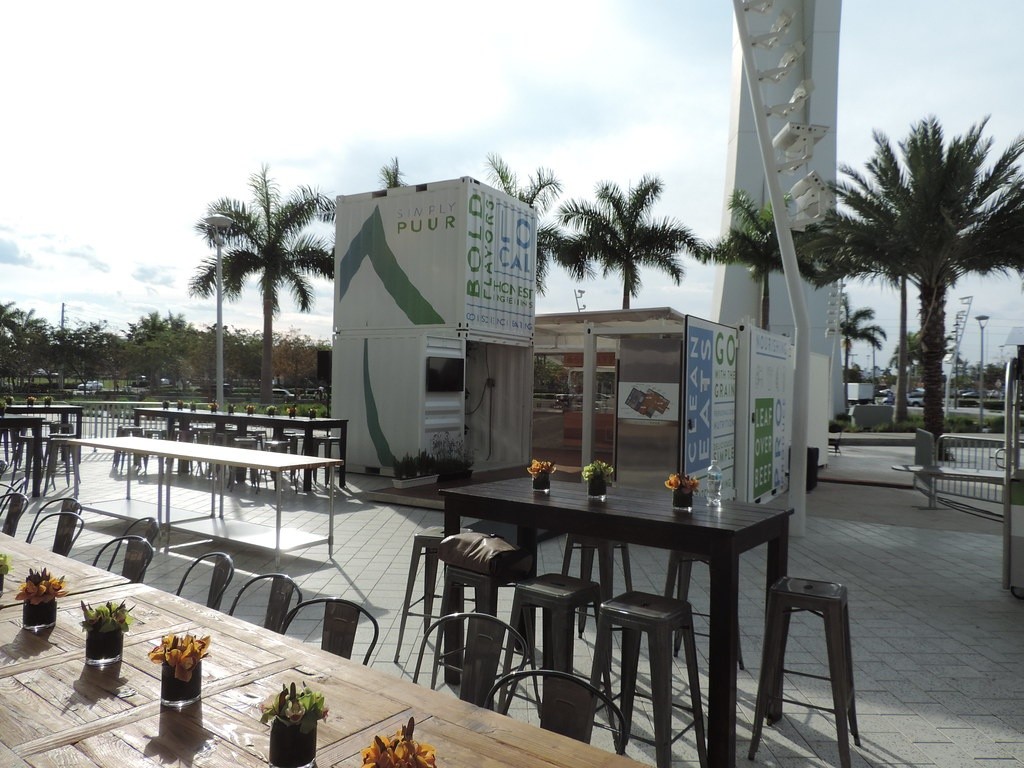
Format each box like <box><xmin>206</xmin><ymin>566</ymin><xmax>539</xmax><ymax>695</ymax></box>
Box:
<box><xmin>431</xmin><ymin>431</ymin><xmax>479</xmax><ymax>473</ymax></box>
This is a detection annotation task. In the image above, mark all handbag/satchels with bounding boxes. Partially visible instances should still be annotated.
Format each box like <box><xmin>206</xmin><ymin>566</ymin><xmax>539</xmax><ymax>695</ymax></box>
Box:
<box><xmin>439</xmin><ymin>532</ymin><xmax>533</xmax><ymax>578</ymax></box>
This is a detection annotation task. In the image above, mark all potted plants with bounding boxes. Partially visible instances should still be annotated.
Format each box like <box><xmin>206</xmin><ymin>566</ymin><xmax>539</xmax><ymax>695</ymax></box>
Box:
<box><xmin>26</xmin><ymin>396</ymin><xmax>36</xmax><ymax>406</ymax></box>
<box><xmin>44</xmin><ymin>396</ymin><xmax>53</xmax><ymax>406</ymax></box>
<box><xmin>81</xmin><ymin>596</ymin><xmax>137</xmax><ymax>665</ymax></box>
<box><xmin>0</xmin><ymin>402</ymin><xmax>7</xmax><ymax>416</ymax></box>
<box><xmin>163</xmin><ymin>400</ymin><xmax>318</xmax><ymax>419</ymax></box>
<box><xmin>582</xmin><ymin>459</ymin><xmax>614</xmax><ymax>498</ymax></box>
<box><xmin>528</xmin><ymin>458</ymin><xmax>555</xmax><ymax>491</ymax></box>
<box><xmin>391</xmin><ymin>450</ymin><xmax>440</xmax><ymax>489</ymax></box>
<box><xmin>147</xmin><ymin>629</ymin><xmax>212</xmax><ymax>708</ymax></box>
<box><xmin>0</xmin><ymin>554</ymin><xmax>13</xmax><ymax>592</ymax></box>
<box><xmin>257</xmin><ymin>681</ymin><xmax>332</xmax><ymax>768</ymax></box>
<box><xmin>662</xmin><ymin>472</ymin><xmax>698</xmax><ymax>515</ymax></box>
<box><xmin>14</xmin><ymin>567</ymin><xmax>67</xmax><ymax>630</ymax></box>
<box><xmin>4</xmin><ymin>395</ymin><xmax>15</xmax><ymax>405</ymax></box>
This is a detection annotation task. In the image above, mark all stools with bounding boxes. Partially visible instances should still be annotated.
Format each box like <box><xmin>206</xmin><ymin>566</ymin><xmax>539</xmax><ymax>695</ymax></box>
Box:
<box><xmin>0</xmin><ymin>422</ymin><xmax>87</xmax><ymax>494</ymax></box>
<box><xmin>393</xmin><ymin>527</ymin><xmax>863</xmax><ymax>768</ymax></box>
<box><xmin>114</xmin><ymin>426</ymin><xmax>347</xmax><ymax>494</ymax></box>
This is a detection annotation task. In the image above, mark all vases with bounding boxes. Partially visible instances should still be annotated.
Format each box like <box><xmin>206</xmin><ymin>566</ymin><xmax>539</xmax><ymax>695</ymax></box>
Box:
<box><xmin>438</xmin><ymin>469</ymin><xmax>474</xmax><ymax>481</ymax></box>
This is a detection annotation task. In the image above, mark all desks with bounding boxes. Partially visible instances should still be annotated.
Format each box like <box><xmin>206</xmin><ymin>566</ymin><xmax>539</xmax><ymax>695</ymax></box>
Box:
<box><xmin>891</xmin><ymin>464</ymin><xmax>1005</xmax><ymax>513</ymax></box>
<box><xmin>133</xmin><ymin>407</ymin><xmax>349</xmax><ymax>494</ymax></box>
<box><xmin>0</xmin><ymin>416</ymin><xmax>45</xmax><ymax>496</ymax></box>
<box><xmin>0</xmin><ymin>404</ymin><xmax>85</xmax><ymax>463</ymax></box>
<box><xmin>443</xmin><ymin>483</ymin><xmax>794</xmax><ymax>768</ymax></box>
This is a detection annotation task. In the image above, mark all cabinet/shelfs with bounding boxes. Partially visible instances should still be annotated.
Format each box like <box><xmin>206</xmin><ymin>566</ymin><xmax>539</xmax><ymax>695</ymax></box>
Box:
<box><xmin>164</xmin><ymin>443</ymin><xmax>343</xmax><ymax>571</ymax></box>
<box><xmin>51</xmin><ymin>435</ymin><xmax>174</xmax><ymax>544</ymax></box>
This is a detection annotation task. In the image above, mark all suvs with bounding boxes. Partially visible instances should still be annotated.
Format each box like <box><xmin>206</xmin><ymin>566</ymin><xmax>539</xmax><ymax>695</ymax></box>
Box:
<box><xmin>195</xmin><ymin>383</ymin><xmax>232</xmax><ymax>397</ymax></box>
<box><xmin>272</xmin><ymin>388</ymin><xmax>295</xmax><ymax>398</ymax></box>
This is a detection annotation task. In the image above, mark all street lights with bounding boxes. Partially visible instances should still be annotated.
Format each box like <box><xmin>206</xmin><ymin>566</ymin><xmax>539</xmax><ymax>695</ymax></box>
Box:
<box><xmin>974</xmin><ymin>315</ymin><xmax>989</xmax><ymax>432</ymax></box>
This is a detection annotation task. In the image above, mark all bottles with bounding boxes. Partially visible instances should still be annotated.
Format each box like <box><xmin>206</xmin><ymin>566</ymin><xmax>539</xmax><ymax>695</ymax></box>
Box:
<box><xmin>706</xmin><ymin>459</ymin><xmax>722</xmax><ymax>507</ymax></box>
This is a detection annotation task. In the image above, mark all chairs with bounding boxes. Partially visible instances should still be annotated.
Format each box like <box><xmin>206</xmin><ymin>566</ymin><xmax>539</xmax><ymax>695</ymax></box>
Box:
<box><xmin>0</xmin><ymin>486</ymin><xmax>627</xmax><ymax>768</ymax></box>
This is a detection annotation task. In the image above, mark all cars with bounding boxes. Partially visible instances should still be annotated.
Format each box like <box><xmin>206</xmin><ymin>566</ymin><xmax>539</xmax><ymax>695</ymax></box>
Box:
<box><xmin>878</xmin><ymin>387</ymin><xmax>925</xmax><ymax>407</ymax></box>
<box><xmin>78</xmin><ymin>381</ymin><xmax>103</xmax><ymax>391</ymax></box>
<box><xmin>957</xmin><ymin>390</ymin><xmax>1005</xmax><ymax>398</ymax></box>
